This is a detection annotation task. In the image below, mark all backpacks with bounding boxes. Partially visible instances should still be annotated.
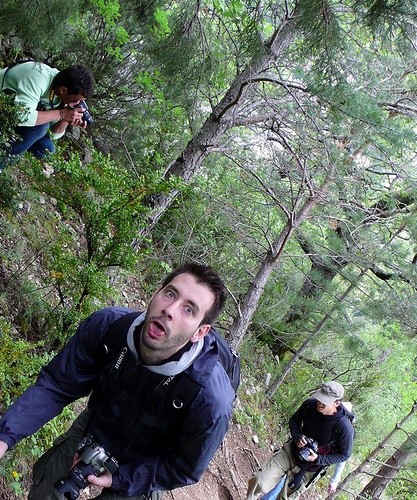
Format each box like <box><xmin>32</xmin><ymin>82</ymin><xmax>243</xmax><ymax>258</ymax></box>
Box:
<box><xmin>103</xmin><ymin>312</ymin><xmax>240</xmax><ymax>410</ymax></box>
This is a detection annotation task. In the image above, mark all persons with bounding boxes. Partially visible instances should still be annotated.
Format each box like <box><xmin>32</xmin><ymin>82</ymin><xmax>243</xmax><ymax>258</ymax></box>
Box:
<box><xmin>0</xmin><ymin>61</ymin><xmax>97</xmax><ymax>170</ymax></box>
<box><xmin>247</xmin><ymin>380</ymin><xmax>354</xmax><ymax>500</ymax></box>
<box><xmin>0</xmin><ymin>262</ymin><xmax>239</xmax><ymax>500</ymax></box>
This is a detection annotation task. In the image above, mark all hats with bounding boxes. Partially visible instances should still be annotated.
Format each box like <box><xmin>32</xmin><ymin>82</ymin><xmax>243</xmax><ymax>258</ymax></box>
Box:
<box><xmin>314</xmin><ymin>381</ymin><xmax>344</xmax><ymax>405</ymax></box>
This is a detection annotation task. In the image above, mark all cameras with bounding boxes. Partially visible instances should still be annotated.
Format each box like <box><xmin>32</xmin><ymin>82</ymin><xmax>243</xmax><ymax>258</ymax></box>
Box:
<box><xmin>298</xmin><ymin>438</ymin><xmax>319</xmax><ymax>461</ymax></box>
<box><xmin>52</xmin><ymin>434</ymin><xmax>120</xmax><ymax>500</ymax></box>
<box><xmin>73</xmin><ymin>101</ymin><xmax>93</xmax><ymax>126</ymax></box>
